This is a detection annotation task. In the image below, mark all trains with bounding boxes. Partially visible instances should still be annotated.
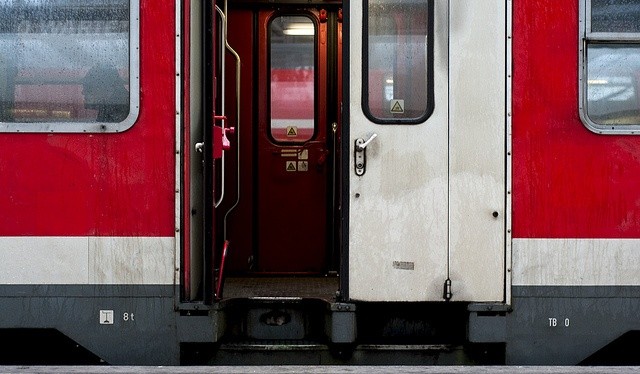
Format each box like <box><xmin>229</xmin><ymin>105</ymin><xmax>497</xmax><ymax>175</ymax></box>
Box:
<box><xmin>0</xmin><ymin>0</ymin><xmax>639</xmax><ymax>366</ymax></box>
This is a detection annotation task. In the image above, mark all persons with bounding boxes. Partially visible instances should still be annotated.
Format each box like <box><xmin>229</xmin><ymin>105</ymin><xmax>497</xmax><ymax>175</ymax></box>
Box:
<box><xmin>83</xmin><ymin>65</ymin><xmax>129</xmax><ymax>123</ymax></box>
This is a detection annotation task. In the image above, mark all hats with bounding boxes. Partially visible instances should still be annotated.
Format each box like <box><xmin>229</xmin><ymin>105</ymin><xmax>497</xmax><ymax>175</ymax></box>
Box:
<box><xmin>78</xmin><ymin>67</ymin><xmax>124</xmax><ymax>84</ymax></box>
